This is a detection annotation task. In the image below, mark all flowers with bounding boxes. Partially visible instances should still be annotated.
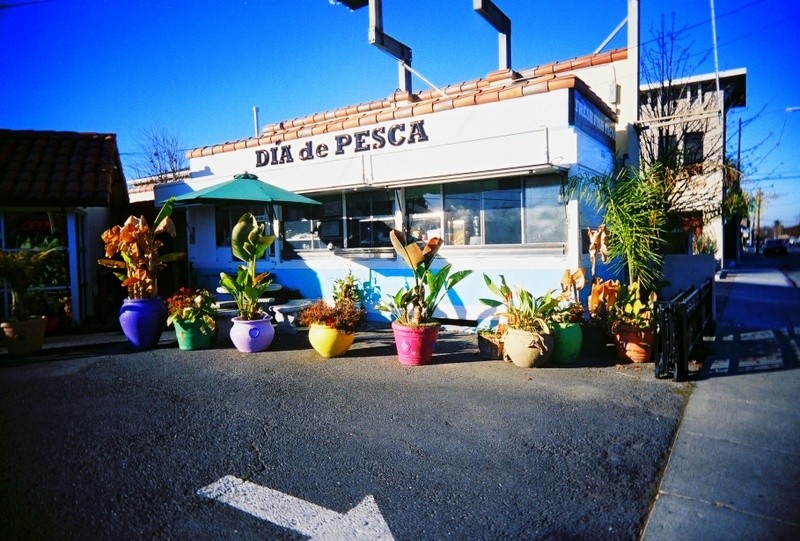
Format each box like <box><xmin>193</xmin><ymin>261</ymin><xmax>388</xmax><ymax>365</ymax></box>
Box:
<box><xmin>166</xmin><ymin>285</ymin><xmax>217</xmax><ymax>331</ymax></box>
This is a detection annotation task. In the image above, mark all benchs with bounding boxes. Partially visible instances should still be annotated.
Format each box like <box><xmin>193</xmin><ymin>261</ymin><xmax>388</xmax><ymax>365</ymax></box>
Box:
<box><xmin>210</xmin><ymin>297</ymin><xmax>275</xmax><ymax>339</ymax></box>
<box><xmin>271</xmin><ymin>299</ymin><xmax>311</xmax><ymax>334</ymax></box>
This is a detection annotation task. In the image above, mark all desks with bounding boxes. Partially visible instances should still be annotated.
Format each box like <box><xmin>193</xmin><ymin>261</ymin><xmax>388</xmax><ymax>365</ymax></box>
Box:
<box><xmin>216</xmin><ymin>283</ymin><xmax>282</xmax><ymax>294</ymax></box>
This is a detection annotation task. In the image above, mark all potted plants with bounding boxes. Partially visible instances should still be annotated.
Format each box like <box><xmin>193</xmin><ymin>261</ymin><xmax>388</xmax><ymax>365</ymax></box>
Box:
<box><xmin>476</xmin><ymin>270</ymin><xmax>608</xmax><ymax>367</ymax></box>
<box><xmin>557</xmin><ymin>153</ymin><xmax>677</xmax><ymax>363</ymax></box>
<box><xmin>97</xmin><ymin>195</ymin><xmax>177</xmax><ymax>352</ymax></box>
<box><xmin>173</xmin><ymin>320</ymin><xmax>211</xmax><ymax>350</ymax></box>
<box><xmin>219</xmin><ymin>212</ymin><xmax>276</xmax><ymax>352</ymax></box>
<box><xmin>0</xmin><ymin>233</ymin><xmax>69</xmax><ymax>356</ymax></box>
<box><xmin>373</xmin><ymin>229</ymin><xmax>473</xmax><ymax>365</ymax></box>
<box><xmin>296</xmin><ymin>270</ymin><xmax>367</xmax><ymax>358</ymax></box>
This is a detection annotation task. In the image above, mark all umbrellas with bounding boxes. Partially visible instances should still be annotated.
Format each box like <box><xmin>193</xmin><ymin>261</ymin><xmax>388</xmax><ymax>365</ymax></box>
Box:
<box><xmin>154</xmin><ymin>171</ymin><xmax>325</xmax><ymax>283</ymax></box>
